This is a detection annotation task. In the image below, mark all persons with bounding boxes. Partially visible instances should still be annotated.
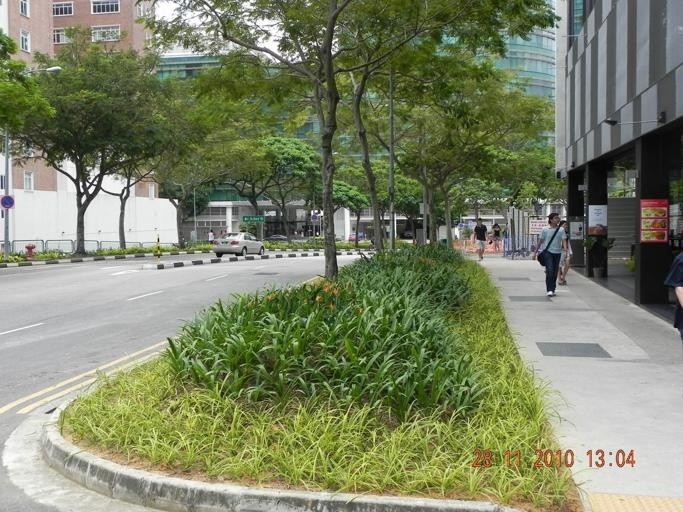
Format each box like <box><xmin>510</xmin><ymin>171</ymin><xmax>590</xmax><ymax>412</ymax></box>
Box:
<box><xmin>206</xmin><ymin>229</ymin><xmax>215</xmax><ymax>244</ymax></box>
<box><xmin>531</xmin><ymin>212</ymin><xmax>568</xmax><ymax>297</ymax></box>
<box><xmin>662</xmin><ymin>251</ymin><xmax>683</xmax><ymax>346</ymax></box>
<box><xmin>490</xmin><ymin>223</ymin><xmax>502</xmax><ymax>252</ymax></box>
<box><xmin>553</xmin><ymin>220</ymin><xmax>573</xmax><ymax>286</ymax></box>
<box><xmin>472</xmin><ymin>217</ymin><xmax>488</xmax><ymax>261</ymax></box>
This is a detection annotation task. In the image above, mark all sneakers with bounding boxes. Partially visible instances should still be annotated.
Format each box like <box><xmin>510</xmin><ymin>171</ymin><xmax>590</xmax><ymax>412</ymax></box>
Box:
<box><xmin>547</xmin><ymin>290</ymin><xmax>553</xmax><ymax>296</ymax></box>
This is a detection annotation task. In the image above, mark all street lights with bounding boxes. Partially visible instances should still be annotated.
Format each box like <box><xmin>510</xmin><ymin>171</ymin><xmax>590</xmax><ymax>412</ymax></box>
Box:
<box><xmin>3</xmin><ymin>66</ymin><xmax>62</xmax><ymax>257</ymax></box>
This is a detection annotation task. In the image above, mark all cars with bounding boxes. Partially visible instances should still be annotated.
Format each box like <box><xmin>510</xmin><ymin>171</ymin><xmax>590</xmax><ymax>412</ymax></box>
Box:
<box><xmin>349</xmin><ymin>232</ymin><xmax>363</xmax><ymax>241</ymax></box>
<box><xmin>402</xmin><ymin>231</ymin><xmax>414</xmax><ymax>238</ymax></box>
<box><xmin>266</xmin><ymin>234</ymin><xmax>287</xmax><ymax>242</ymax></box>
<box><xmin>211</xmin><ymin>232</ymin><xmax>265</xmax><ymax>257</ymax></box>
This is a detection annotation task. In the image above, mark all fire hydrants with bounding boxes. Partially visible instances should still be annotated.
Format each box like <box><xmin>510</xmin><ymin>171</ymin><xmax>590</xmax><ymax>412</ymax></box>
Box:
<box><xmin>25</xmin><ymin>244</ymin><xmax>36</xmax><ymax>260</ymax></box>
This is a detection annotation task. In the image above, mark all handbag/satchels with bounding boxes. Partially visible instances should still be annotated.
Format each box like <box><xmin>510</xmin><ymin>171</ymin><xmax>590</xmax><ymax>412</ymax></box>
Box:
<box><xmin>538</xmin><ymin>249</ymin><xmax>548</xmax><ymax>266</ymax></box>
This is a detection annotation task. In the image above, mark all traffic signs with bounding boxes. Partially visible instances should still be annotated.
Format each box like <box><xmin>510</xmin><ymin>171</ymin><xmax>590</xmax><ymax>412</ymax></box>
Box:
<box><xmin>242</xmin><ymin>216</ymin><xmax>266</xmax><ymax>223</ymax></box>
